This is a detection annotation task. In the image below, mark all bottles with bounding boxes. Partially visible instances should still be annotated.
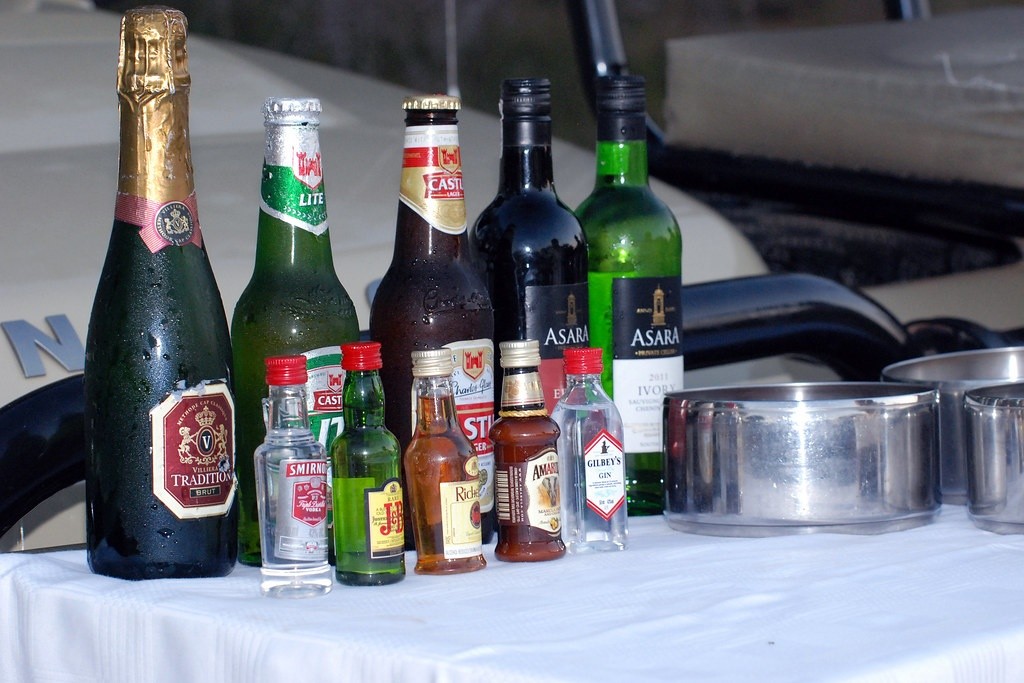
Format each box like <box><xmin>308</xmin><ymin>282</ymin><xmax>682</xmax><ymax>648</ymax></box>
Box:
<box><xmin>231</xmin><ymin>97</ymin><xmax>360</xmax><ymax>566</ymax></box>
<box><xmin>489</xmin><ymin>339</ymin><xmax>566</xmax><ymax>561</ymax></box>
<box><xmin>552</xmin><ymin>348</ymin><xmax>628</xmax><ymax>554</ymax></box>
<box><xmin>331</xmin><ymin>342</ymin><xmax>405</xmax><ymax>585</ymax></box>
<box><xmin>83</xmin><ymin>8</ymin><xmax>238</xmax><ymax>580</ymax></box>
<box><xmin>575</xmin><ymin>75</ymin><xmax>682</xmax><ymax>516</ymax></box>
<box><xmin>404</xmin><ymin>347</ymin><xmax>486</xmax><ymax>575</ymax></box>
<box><xmin>469</xmin><ymin>78</ymin><xmax>590</xmax><ymax>531</ymax></box>
<box><xmin>370</xmin><ymin>94</ymin><xmax>494</xmax><ymax>550</ymax></box>
<box><xmin>253</xmin><ymin>354</ymin><xmax>332</xmax><ymax>600</ymax></box>
